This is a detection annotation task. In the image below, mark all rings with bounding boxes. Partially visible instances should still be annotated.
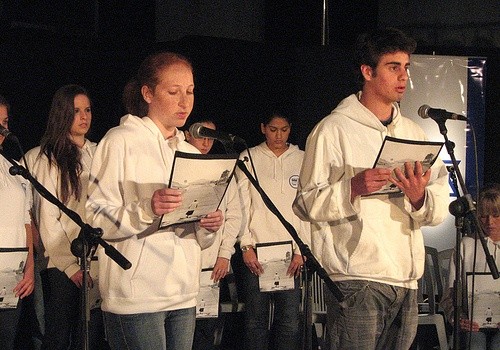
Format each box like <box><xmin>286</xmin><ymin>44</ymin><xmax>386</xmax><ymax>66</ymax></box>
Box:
<box><xmin>218</xmin><ymin>270</ymin><xmax>221</xmax><ymax>272</ymax></box>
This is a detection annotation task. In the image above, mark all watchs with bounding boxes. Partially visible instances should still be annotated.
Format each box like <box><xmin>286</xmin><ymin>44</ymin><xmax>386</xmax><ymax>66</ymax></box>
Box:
<box><xmin>241</xmin><ymin>245</ymin><xmax>252</xmax><ymax>252</ymax></box>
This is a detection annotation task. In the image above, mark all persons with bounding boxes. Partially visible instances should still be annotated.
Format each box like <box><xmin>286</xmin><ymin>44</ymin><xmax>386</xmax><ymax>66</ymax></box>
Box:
<box><xmin>486</xmin><ymin>307</ymin><xmax>493</xmax><ymax>325</ymax></box>
<box><xmin>0</xmin><ymin>287</ymin><xmax>6</xmax><ymax>305</ymax></box>
<box><xmin>85</xmin><ymin>50</ymin><xmax>223</xmax><ymax>350</ymax></box>
<box><xmin>448</xmin><ymin>189</ymin><xmax>500</xmax><ymax>350</ymax></box>
<box><xmin>199</xmin><ymin>299</ymin><xmax>205</xmax><ymax>314</ymax></box>
<box><xmin>235</xmin><ymin>109</ymin><xmax>310</xmax><ymax>350</ymax></box>
<box><xmin>274</xmin><ymin>273</ymin><xmax>280</xmax><ymax>287</ymax></box>
<box><xmin>185</xmin><ymin>199</ymin><xmax>198</xmax><ymax>218</ymax></box>
<box><xmin>292</xmin><ymin>28</ymin><xmax>450</xmax><ymax>350</ymax></box>
<box><xmin>182</xmin><ymin>112</ymin><xmax>243</xmax><ymax>350</ymax></box>
<box><xmin>19</xmin><ymin>85</ymin><xmax>97</xmax><ymax>350</ymax></box>
<box><xmin>0</xmin><ymin>98</ymin><xmax>35</xmax><ymax>350</ymax></box>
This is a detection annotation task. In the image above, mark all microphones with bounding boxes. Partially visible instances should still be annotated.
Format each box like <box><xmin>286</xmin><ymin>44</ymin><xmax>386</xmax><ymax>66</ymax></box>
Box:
<box><xmin>0</xmin><ymin>124</ymin><xmax>12</xmax><ymax>139</ymax></box>
<box><xmin>418</xmin><ymin>104</ymin><xmax>469</xmax><ymax>121</ymax></box>
<box><xmin>189</xmin><ymin>124</ymin><xmax>246</xmax><ymax>144</ymax></box>
